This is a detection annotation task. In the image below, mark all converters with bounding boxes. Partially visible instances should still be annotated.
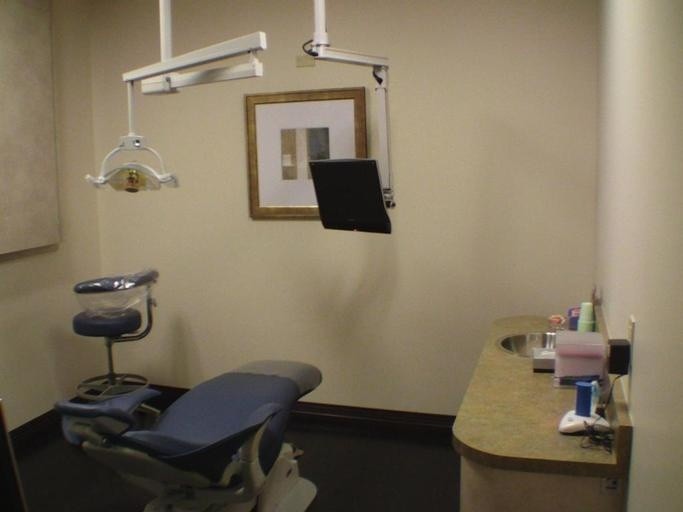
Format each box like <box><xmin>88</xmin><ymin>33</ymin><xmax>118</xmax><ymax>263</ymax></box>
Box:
<box><xmin>607</xmin><ymin>340</ymin><xmax>628</xmax><ymax>375</ymax></box>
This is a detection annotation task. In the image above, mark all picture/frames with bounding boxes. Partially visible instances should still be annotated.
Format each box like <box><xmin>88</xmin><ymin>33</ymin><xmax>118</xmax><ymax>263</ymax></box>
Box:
<box><xmin>245</xmin><ymin>88</ymin><xmax>367</xmax><ymax>219</ymax></box>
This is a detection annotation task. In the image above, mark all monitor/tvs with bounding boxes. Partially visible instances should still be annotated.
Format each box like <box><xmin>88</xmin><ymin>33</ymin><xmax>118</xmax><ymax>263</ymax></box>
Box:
<box><xmin>307</xmin><ymin>159</ymin><xmax>392</xmax><ymax>235</ymax></box>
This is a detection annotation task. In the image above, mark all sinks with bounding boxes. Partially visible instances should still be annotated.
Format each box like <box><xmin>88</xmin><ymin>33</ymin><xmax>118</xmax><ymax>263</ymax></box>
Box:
<box><xmin>497</xmin><ymin>329</ymin><xmax>556</xmax><ymax>358</ymax></box>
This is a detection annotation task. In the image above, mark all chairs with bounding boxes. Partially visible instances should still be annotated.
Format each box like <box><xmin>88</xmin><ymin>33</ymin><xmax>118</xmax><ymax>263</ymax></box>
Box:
<box><xmin>53</xmin><ymin>358</ymin><xmax>327</xmax><ymax>512</ymax></box>
<box><xmin>72</xmin><ymin>269</ymin><xmax>159</xmax><ymax>401</ymax></box>
